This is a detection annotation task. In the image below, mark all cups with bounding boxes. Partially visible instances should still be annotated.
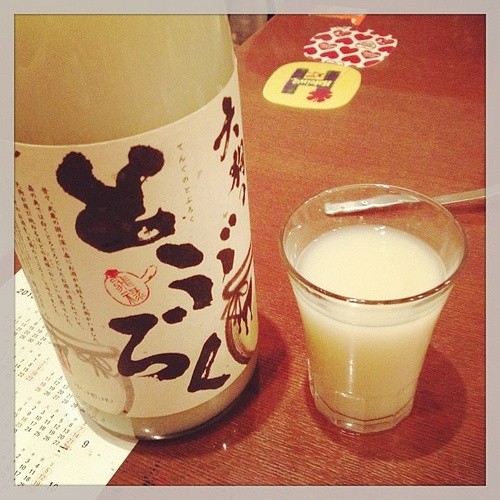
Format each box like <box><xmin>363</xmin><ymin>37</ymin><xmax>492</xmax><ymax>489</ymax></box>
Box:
<box><xmin>278</xmin><ymin>184</ymin><xmax>469</xmax><ymax>435</ymax></box>
<box><xmin>231</xmin><ymin>14</ymin><xmax>268</xmax><ymax>44</ymax></box>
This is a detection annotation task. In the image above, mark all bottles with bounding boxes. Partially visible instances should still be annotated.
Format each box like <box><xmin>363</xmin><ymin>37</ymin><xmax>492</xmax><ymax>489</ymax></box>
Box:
<box><xmin>14</xmin><ymin>14</ymin><xmax>260</xmax><ymax>440</ymax></box>
